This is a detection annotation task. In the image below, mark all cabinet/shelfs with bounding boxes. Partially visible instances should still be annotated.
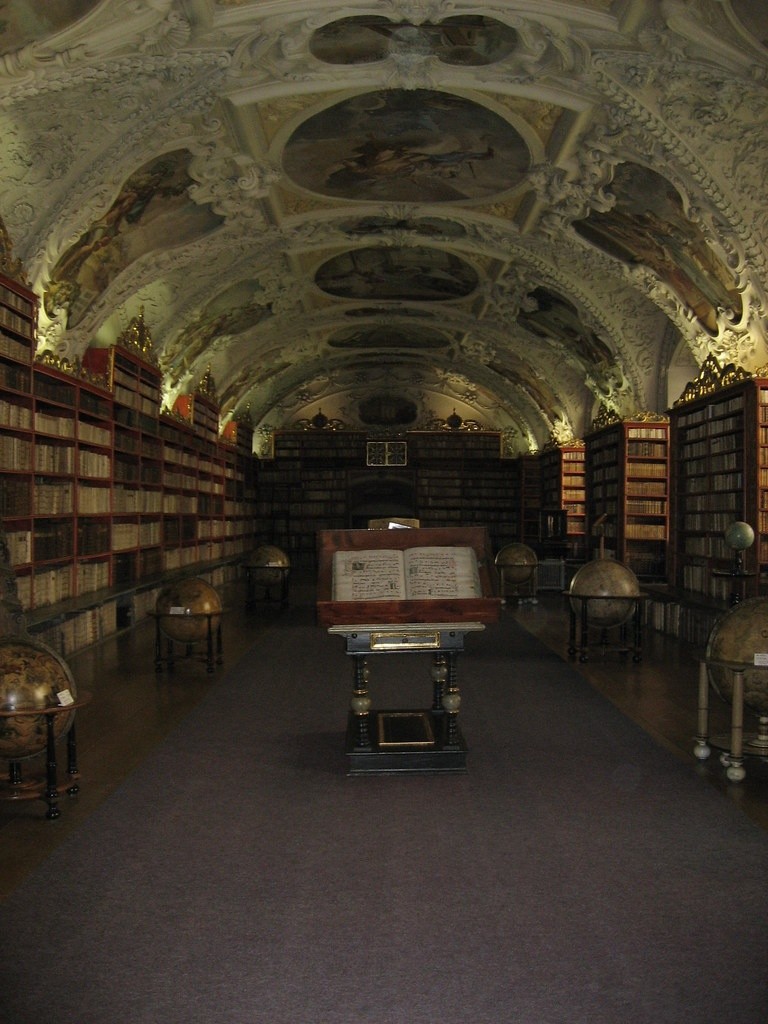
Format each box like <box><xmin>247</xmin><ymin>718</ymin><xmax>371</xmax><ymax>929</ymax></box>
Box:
<box><xmin>0</xmin><ymin>274</ymin><xmax>768</xmax><ymax>662</ymax></box>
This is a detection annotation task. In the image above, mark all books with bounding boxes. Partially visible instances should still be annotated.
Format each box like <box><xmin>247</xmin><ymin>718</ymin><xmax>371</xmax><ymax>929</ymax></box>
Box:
<box><xmin>257</xmin><ymin>428</ymin><xmax>668</xmax><ymax>583</ymax></box>
<box><xmin>332</xmin><ymin>546</ymin><xmax>481</xmax><ymax>601</ymax></box>
<box><xmin>642</xmin><ymin>390</ymin><xmax>768</xmax><ymax>664</ymax></box>
<box><xmin>0</xmin><ymin>285</ymin><xmax>257</xmax><ymax>656</ymax></box>
